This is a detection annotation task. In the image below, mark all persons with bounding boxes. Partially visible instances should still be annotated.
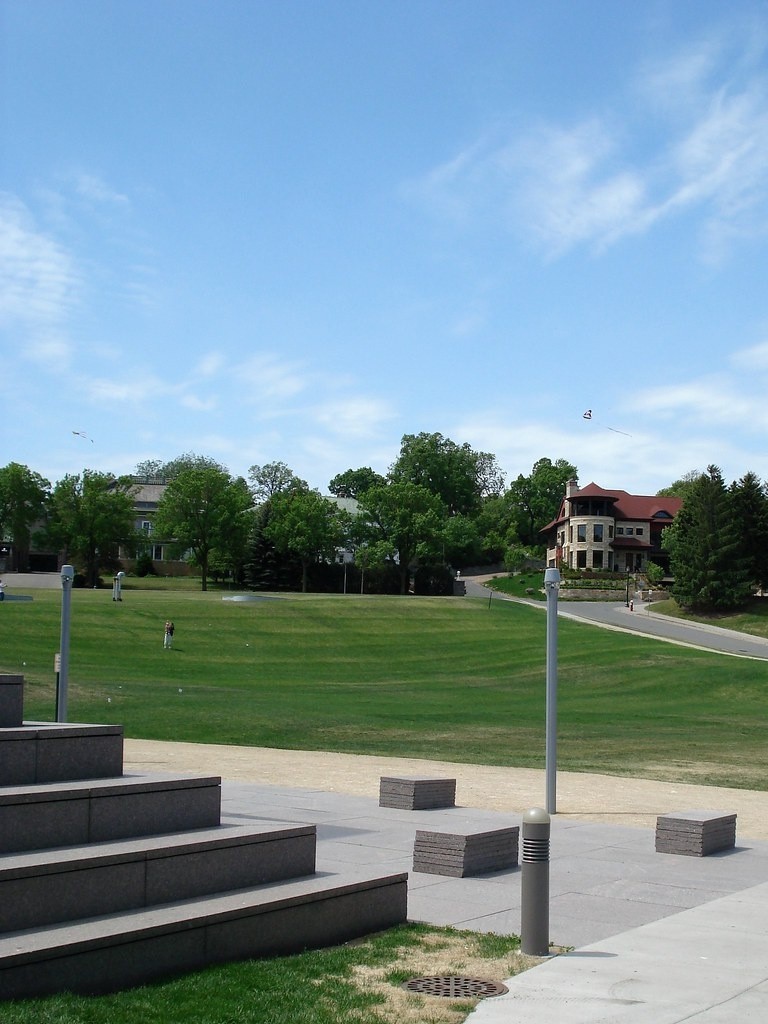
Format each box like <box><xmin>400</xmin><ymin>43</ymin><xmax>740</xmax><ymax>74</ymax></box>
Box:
<box><xmin>164</xmin><ymin>618</ymin><xmax>174</xmax><ymax>650</ymax></box>
<box><xmin>0</xmin><ymin>579</ymin><xmax>7</xmax><ymax>600</ymax></box>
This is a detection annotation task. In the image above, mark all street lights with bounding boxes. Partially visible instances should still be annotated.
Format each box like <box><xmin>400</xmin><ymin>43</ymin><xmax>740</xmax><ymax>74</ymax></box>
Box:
<box><xmin>58</xmin><ymin>565</ymin><xmax>74</xmax><ymax>723</ymax></box>
<box><xmin>543</xmin><ymin>567</ymin><xmax>560</xmax><ymax>815</ymax></box>
<box><xmin>625</xmin><ymin>566</ymin><xmax>630</xmax><ymax>606</ymax></box>
